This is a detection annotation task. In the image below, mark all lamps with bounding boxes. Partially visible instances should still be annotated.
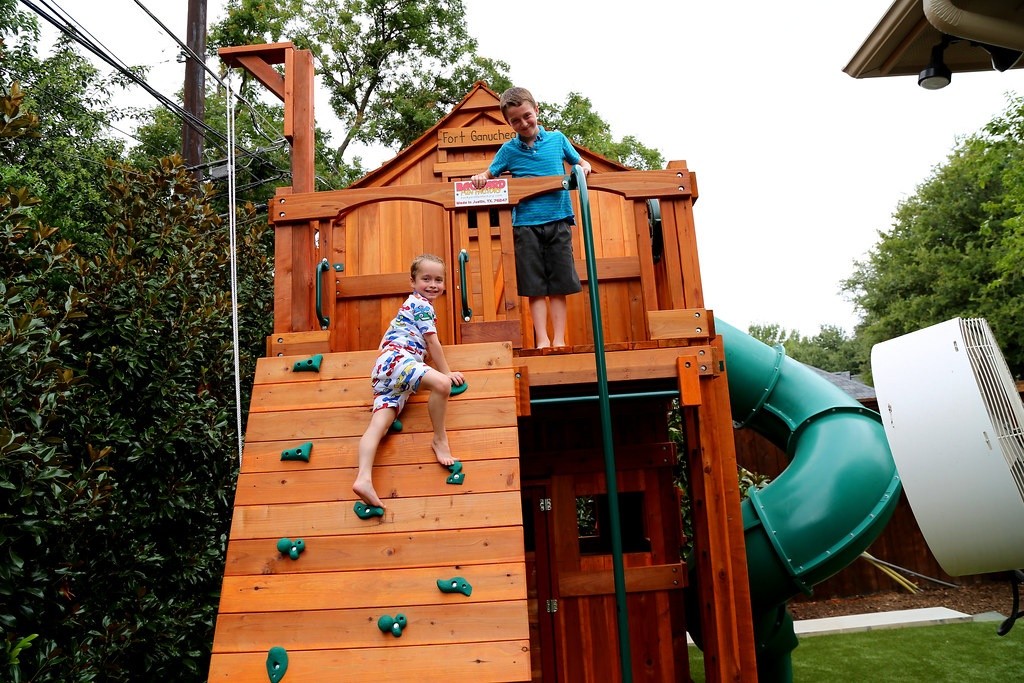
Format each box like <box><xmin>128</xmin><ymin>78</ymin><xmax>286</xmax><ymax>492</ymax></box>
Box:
<box><xmin>917</xmin><ymin>31</ymin><xmax>1024</xmax><ymax>90</ymax></box>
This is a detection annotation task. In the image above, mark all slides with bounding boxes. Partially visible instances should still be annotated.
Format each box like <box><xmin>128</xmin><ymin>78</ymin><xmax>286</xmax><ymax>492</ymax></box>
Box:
<box><xmin>685</xmin><ymin>310</ymin><xmax>904</xmax><ymax>683</ymax></box>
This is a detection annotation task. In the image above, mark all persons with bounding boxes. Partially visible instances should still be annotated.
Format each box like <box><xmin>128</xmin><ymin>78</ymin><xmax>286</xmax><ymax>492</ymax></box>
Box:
<box><xmin>470</xmin><ymin>87</ymin><xmax>592</xmax><ymax>350</ymax></box>
<box><xmin>352</xmin><ymin>254</ymin><xmax>466</xmax><ymax>509</ymax></box>
